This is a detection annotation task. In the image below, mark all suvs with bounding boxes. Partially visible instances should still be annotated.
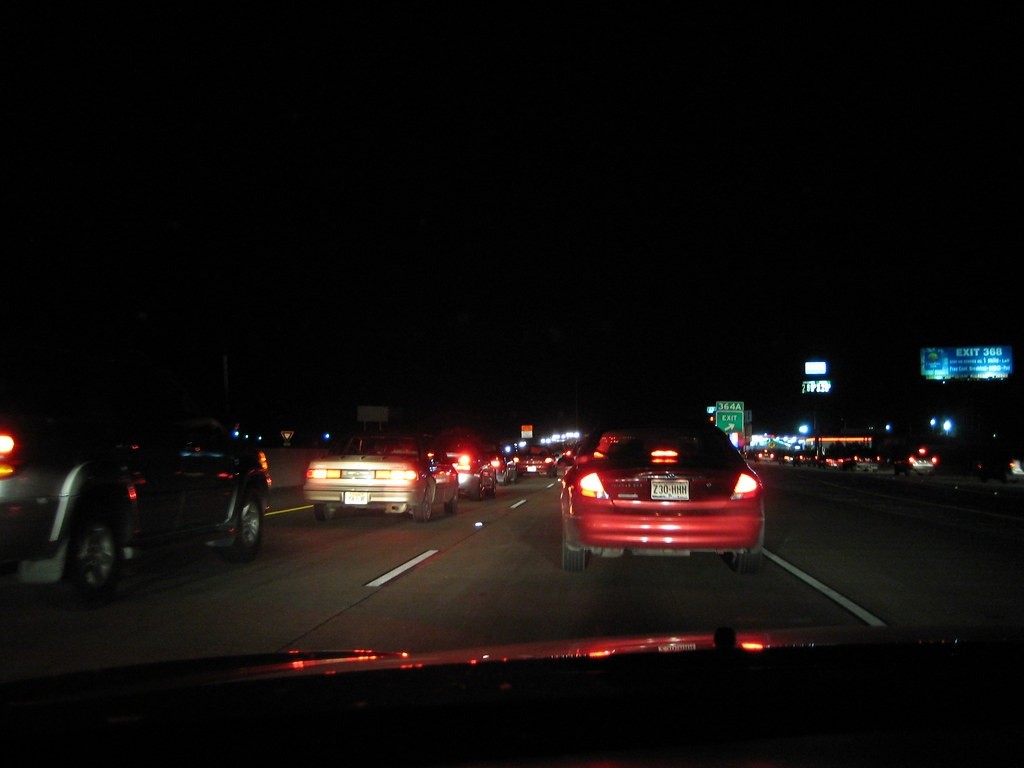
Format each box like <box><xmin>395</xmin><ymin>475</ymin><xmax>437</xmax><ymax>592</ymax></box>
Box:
<box><xmin>0</xmin><ymin>399</ymin><xmax>274</xmax><ymax>607</ymax></box>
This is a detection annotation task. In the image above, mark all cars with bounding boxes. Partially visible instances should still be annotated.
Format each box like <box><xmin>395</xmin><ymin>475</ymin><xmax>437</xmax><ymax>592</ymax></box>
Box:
<box><xmin>752</xmin><ymin>435</ymin><xmax>940</xmax><ymax>477</ymax></box>
<box><xmin>299</xmin><ymin>429</ymin><xmax>586</xmax><ymax>524</ymax></box>
<box><xmin>556</xmin><ymin>415</ymin><xmax>766</xmax><ymax>575</ymax></box>
